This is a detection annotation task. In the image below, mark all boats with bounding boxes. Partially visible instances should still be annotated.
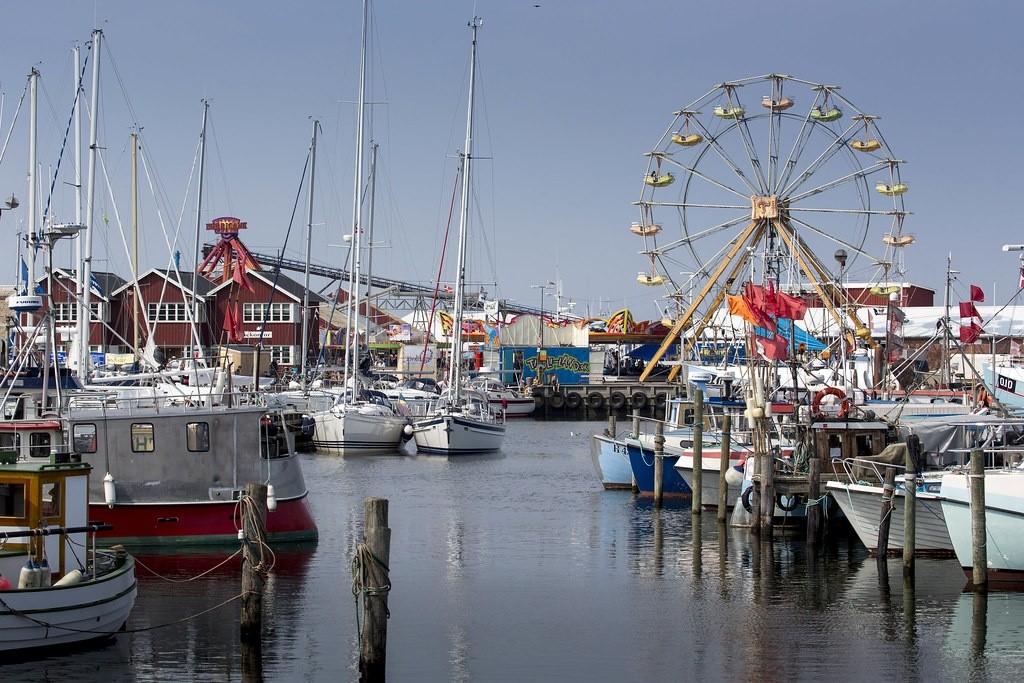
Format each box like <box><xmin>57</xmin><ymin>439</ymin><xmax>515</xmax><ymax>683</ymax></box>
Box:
<box><xmin>375</xmin><ymin>373</ymin><xmax>537</xmax><ymax>416</ymax></box>
<box><xmin>588</xmin><ymin>243</ymin><xmax>1024</xmax><ymax>593</ymax></box>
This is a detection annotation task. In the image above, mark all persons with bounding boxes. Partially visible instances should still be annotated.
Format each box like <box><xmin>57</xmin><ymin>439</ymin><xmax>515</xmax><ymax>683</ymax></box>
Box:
<box><xmin>532</xmin><ymin>376</ymin><xmax>539</xmax><ymax>387</ymax></box>
<box><xmin>551</xmin><ymin>374</ymin><xmax>560</xmax><ymax>392</ymax></box>
<box><xmin>611</xmin><ymin>351</ymin><xmax>644</xmax><ymax>374</ymax></box>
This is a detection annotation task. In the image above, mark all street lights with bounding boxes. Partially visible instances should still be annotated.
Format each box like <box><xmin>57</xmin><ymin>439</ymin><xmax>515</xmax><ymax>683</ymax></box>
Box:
<box><xmin>680</xmin><ymin>269</ymin><xmax>694</xmax><ymax>328</ymax></box>
<box><xmin>532</xmin><ymin>283</ymin><xmax>554</xmax><ymax>349</ymax></box>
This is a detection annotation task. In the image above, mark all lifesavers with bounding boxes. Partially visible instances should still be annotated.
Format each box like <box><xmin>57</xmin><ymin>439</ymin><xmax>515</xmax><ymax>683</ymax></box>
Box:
<box><xmin>549</xmin><ymin>392</ymin><xmax>565</xmax><ymax>408</ymax></box>
<box><xmin>630</xmin><ymin>392</ymin><xmax>647</xmax><ymax>409</ymax></box>
<box><xmin>654</xmin><ymin>392</ymin><xmax>666</xmax><ymax>409</ymax></box>
<box><xmin>586</xmin><ymin>391</ymin><xmax>603</xmax><ymax>409</ymax></box>
<box><xmin>609</xmin><ymin>392</ymin><xmax>625</xmax><ymax>409</ymax></box>
<box><xmin>777</xmin><ymin>493</ymin><xmax>799</xmax><ymax>511</ymax></box>
<box><xmin>565</xmin><ymin>392</ymin><xmax>581</xmax><ymax>409</ymax></box>
<box><xmin>742</xmin><ymin>486</ymin><xmax>753</xmax><ymax>515</ymax></box>
<box><xmin>531</xmin><ymin>391</ymin><xmax>545</xmax><ymax>410</ymax></box>
<box><xmin>812</xmin><ymin>388</ymin><xmax>849</xmax><ymax>418</ymax></box>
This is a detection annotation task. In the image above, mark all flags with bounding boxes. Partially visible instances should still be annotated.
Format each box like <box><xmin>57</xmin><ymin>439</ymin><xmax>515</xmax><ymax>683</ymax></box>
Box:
<box><xmin>726</xmin><ymin>279</ymin><xmax>808</xmax><ymax>363</ymax></box>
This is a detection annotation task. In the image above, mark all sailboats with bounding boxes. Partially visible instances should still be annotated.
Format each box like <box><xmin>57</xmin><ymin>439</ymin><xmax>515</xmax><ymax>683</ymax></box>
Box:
<box><xmin>410</xmin><ymin>0</ymin><xmax>507</xmax><ymax>459</ymax></box>
<box><xmin>314</xmin><ymin>2</ymin><xmax>414</xmax><ymax>456</ymax></box>
<box><xmin>1</xmin><ymin>22</ymin><xmax>327</xmax><ymax>664</ymax></box>
